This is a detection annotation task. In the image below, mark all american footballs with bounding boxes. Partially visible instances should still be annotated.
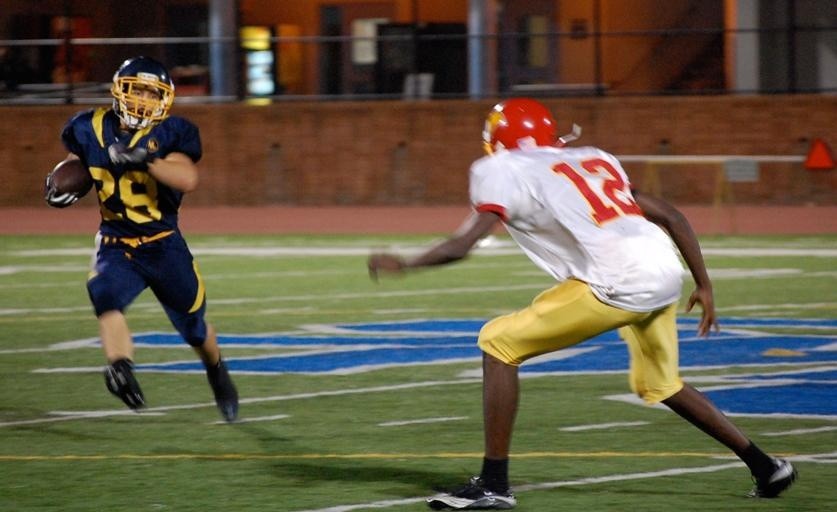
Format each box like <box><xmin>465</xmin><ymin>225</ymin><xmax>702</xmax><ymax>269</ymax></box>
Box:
<box><xmin>51</xmin><ymin>159</ymin><xmax>92</xmax><ymax>199</ymax></box>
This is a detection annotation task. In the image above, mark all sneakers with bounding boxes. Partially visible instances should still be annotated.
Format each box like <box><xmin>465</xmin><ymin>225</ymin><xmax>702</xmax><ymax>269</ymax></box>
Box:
<box><xmin>427</xmin><ymin>477</ymin><xmax>518</xmax><ymax>510</ymax></box>
<box><xmin>101</xmin><ymin>365</ymin><xmax>146</xmax><ymax>409</ymax></box>
<box><xmin>750</xmin><ymin>460</ymin><xmax>799</xmax><ymax>496</ymax></box>
<box><xmin>208</xmin><ymin>367</ymin><xmax>241</xmax><ymax>420</ymax></box>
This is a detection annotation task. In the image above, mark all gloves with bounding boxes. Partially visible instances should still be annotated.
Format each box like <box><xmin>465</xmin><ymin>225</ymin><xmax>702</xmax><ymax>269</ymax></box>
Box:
<box><xmin>44</xmin><ymin>170</ymin><xmax>80</xmax><ymax>207</ymax></box>
<box><xmin>107</xmin><ymin>141</ymin><xmax>154</xmax><ymax>175</ymax></box>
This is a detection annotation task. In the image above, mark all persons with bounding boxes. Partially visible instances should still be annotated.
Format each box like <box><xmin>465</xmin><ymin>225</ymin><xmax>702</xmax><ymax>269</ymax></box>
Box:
<box><xmin>44</xmin><ymin>54</ymin><xmax>238</xmax><ymax>426</ymax></box>
<box><xmin>366</xmin><ymin>98</ymin><xmax>801</xmax><ymax>510</ymax></box>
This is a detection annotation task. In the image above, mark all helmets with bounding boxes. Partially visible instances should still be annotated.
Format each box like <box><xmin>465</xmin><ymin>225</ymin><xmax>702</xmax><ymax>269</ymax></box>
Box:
<box><xmin>112</xmin><ymin>55</ymin><xmax>175</xmax><ymax>129</ymax></box>
<box><xmin>483</xmin><ymin>98</ymin><xmax>560</xmax><ymax>157</ymax></box>
